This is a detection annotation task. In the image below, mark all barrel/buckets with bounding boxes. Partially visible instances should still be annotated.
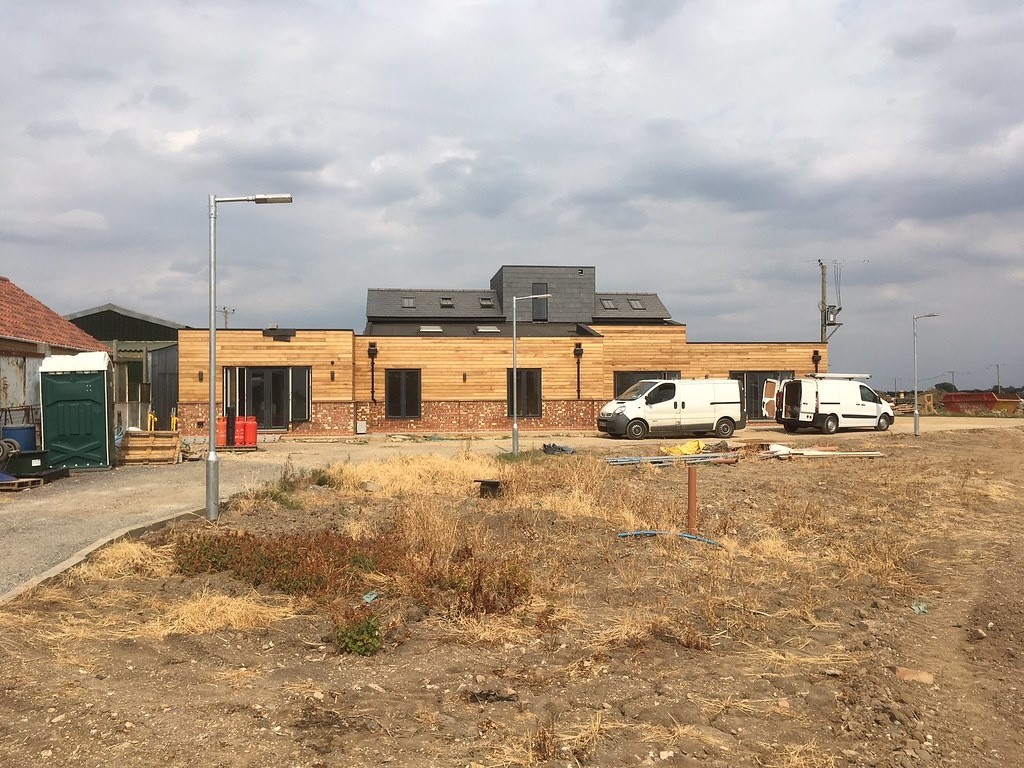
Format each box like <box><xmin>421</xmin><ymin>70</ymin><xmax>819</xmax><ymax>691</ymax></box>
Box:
<box><xmin>2</xmin><ymin>424</ymin><xmax>36</xmax><ymax>451</ymax></box>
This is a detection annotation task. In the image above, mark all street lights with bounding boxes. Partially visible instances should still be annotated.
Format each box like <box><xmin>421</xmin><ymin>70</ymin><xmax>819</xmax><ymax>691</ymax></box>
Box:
<box><xmin>205</xmin><ymin>193</ymin><xmax>294</xmax><ymax>521</ymax></box>
<box><xmin>514</xmin><ymin>293</ymin><xmax>553</xmax><ymax>458</ymax></box>
<box><xmin>914</xmin><ymin>313</ymin><xmax>940</xmax><ymax>436</ymax></box>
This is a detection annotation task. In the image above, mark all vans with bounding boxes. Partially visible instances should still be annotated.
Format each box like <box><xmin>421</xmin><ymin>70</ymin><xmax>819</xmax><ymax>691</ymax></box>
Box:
<box><xmin>762</xmin><ymin>372</ymin><xmax>896</xmax><ymax>433</ymax></box>
<box><xmin>597</xmin><ymin>378</ymin><xmax>746</xmax><ymax>438</ymax></box>
